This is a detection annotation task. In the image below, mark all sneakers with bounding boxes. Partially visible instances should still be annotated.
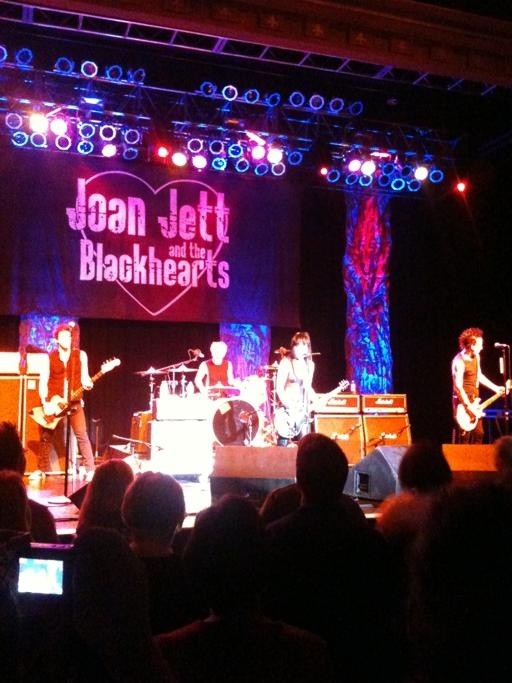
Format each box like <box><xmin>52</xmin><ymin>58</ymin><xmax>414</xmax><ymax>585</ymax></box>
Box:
<box><xmin>86</xmin><ymin>471</ymin><xmax>94</xmax><ymax>481</ymax></box>
<box><xmin>29</xmin><ymin>469</ymin><xmax>45</xmax><ymax>480</ymax></box>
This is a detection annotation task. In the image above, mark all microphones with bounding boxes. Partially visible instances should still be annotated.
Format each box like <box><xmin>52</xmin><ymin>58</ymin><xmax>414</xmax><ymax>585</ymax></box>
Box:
<box><xmin>193</xmin><ymin>350</ymin><xmax>204</xmax><ymax>357</ymax></box>
<box><xmin>494</xmin><ymin>342</ymin><xmax>510</xmax><ymax>348</ymax></box>
<box><xmin>68</xmin><ymin>321</ymin><xmax>76</xmax><ymax>328</ymax></box>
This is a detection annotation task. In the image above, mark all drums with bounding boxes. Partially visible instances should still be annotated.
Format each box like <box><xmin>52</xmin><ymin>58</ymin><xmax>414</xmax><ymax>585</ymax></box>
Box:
<box><xmin>149</xmin><ymin>394</ymin><xmax>186</xmax><ymax>422</ymax></box>
<box><xmin>130</xmin><ymin>411</ymin><xmax>152</xmax><ymax>455</ymax></box>
<box><xmin>206</xmin><ymin>397</ymin><xmax>261</xmax><ymax>446</ymax></box>
<box><xmin>185</xmin><ymin>393</ymin><xmax>215</xmax><ymax>421</ymax></box>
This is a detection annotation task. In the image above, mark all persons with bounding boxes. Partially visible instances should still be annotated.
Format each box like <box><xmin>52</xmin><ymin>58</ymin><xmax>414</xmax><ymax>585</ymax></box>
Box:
<box><xmin>275</xmin><ymin>330</ymin><xmax>325</xmax><ymax>442</ymax></box>
<box><xmin>38</xmin><ymin>324</ymin><xmax>98</xmax><ymax>483</ymax></box>
<box><xmin>194</xmin><ymin>339</ymin><xmax>236</xmax><ymax>393</ymax></box>
<box><xmin>0</xmin><ymin>420</ymin><xmax>512</xmax><ymax>683</ymax></box>
<box><xmin>449</xmin><ymin>326</ymin><xmax>511</xmax><ymax>443</ymax></box>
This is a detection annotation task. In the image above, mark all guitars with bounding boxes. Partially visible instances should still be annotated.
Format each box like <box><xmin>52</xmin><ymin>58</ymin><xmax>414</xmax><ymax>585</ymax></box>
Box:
<box><xmin>457</xmin><ymin>380</ymin><xmax>510</xmax><ymax>432</ymax></box>
<box><xmin>29</xmin><ymin>357</ymin><xmax>121</xmax><ymax>431</ymax></box>
<box><xmin>273</xmin><ymin>379</ymin><xmax>349</xmax><ymax>440</ymax></box>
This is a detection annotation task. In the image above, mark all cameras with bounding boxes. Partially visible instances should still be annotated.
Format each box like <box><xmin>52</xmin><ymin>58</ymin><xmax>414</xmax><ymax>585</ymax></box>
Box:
<box><xmin>18</xmin><ymin>555</ymin><xmax>75</xmax><ymax>598</ymax></box>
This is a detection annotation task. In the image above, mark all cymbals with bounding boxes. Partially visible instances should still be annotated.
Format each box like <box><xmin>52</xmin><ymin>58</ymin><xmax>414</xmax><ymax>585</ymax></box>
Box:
<box><xmin>168</xmin><ymin>367</ymin><xmax>198</xmax><ymax>373</ymax></box>
<box><xmin>135</xmin><ymin>370</ymin><xmax>168</xmax><ymax>376</ymax></box>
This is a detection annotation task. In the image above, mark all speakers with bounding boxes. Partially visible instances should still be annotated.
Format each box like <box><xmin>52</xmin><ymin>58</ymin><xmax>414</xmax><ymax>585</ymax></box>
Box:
<box><xmin>208</xmin><ymin>447</ymin><xmax>297</xmax><ymax>505</ymax></box>
<box><xmin>344</xmin><ymin>445</ymin><xmax>410</xmax><ymax>501</ymax></box>
<box><xmin>69</xmin><ymin>480</ymin><xmax>91</xmax><ymax>511</ymax></box>
